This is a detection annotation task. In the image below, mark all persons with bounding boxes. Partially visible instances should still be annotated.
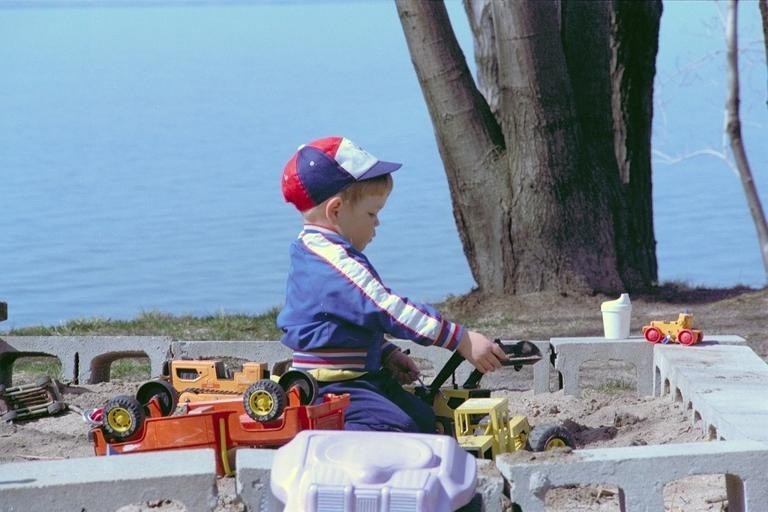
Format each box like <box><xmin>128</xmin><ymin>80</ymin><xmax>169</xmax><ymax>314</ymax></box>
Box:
<box><xmin>274</xmin><ymin>137</ymin><xmax>508</xmax><ymax>432</ymax></box>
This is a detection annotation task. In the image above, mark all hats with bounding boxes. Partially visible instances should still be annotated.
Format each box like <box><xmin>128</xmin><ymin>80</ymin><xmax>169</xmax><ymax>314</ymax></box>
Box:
<box><xmin>281</xmin><ymin>136</ymin><xmax>403</xmax><ymax>211</ymax></box>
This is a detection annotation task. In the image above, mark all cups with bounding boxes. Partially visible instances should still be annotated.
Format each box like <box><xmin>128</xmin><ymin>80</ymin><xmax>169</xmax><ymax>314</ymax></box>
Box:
<box><xmin>601</xmin><ymin>292</ymin><xmax>632</xmax><ymax>341</ymax></box>
